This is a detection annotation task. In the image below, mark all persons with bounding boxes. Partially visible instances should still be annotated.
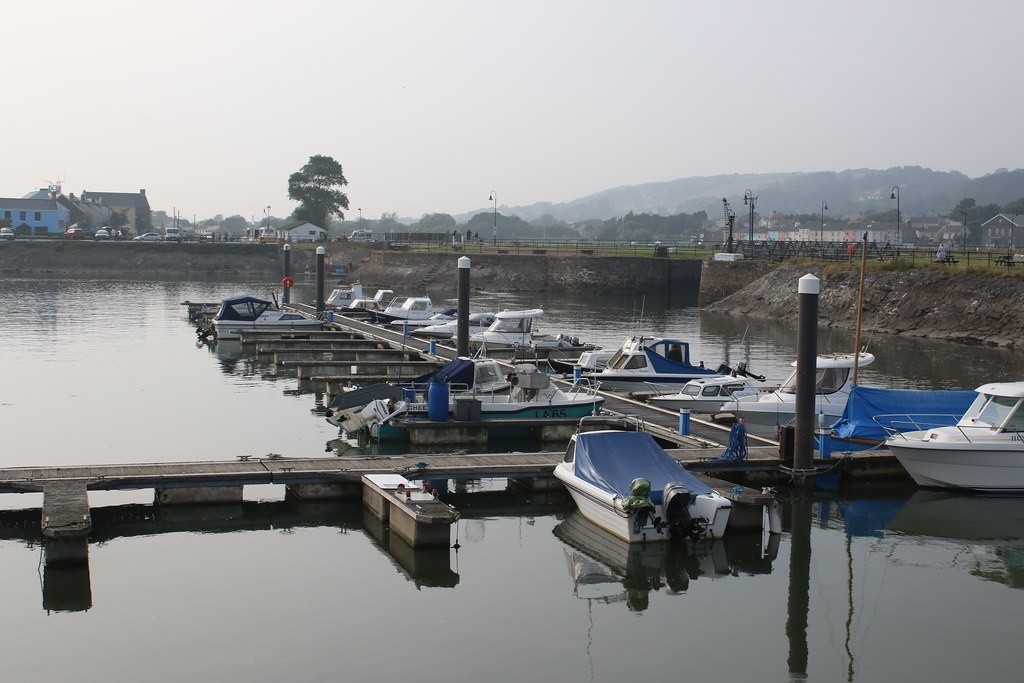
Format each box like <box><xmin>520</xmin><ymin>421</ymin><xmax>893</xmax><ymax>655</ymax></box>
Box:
<box><xmin>666</xmin><ymin>345</ymin><xmax>683</xmax><ymax>364</ymax></box>
<box><xmin>475</xmin><ymin>232</ymin><xmax>478</xmax><ymax>237</ymax></box>
<box><xmin>936</xmin><ymin>244</ymin><xmax>946</xmax><ymax>260</ymax></box>
<box><xmin>467</xmin><ymin>228</ymin><xmax>472</xmax><ymax>241</ymax></box>
<box><xmin>115</xmin><ymin>228</ymin><xmax>350</xmax><ymax>244</ymax></box>
<box><xmin>453</xmin><ymin>230</ymin><xmax>457</xmax><ymax>235</ymax></box>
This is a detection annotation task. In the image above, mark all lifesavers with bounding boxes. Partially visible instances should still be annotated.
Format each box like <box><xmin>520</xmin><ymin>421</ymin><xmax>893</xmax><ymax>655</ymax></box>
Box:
<box><xmin>282</xmin><ymin>276</ymin><xmax>294</xmax><ymax>287</ymax></box>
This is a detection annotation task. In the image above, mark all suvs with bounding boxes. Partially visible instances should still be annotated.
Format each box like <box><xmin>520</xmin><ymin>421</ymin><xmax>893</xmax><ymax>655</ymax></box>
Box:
<box><xmin>163</xmin><ymin>228</ymin><xmax>182</xmax><ymax>242</ymax></box>
<box><xmin>64</xmin><ymin>229</ymin><xmax>84</xmax><ymax>240</ymax></box>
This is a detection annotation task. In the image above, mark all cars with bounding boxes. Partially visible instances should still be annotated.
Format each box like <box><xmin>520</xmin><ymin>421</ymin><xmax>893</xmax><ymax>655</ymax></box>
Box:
<box><xmin>95</xmin><ymin>230</ymin><xmax>109</xmax><ymax>240</ymax></box>
<box><xmin>0</xmin><ymin>228</ymin><xmax>15</xmax><ymax>241</ymax></box>
<box><xmin>134</xmin><ymin>232</ymin><xmax>162</xmax><ymax>242</ymax></box>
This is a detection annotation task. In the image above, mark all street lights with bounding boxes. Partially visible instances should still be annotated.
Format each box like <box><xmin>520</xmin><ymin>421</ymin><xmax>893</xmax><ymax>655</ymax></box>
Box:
<box><xmin>488</xmin><ymin>191</ymin><xmax>496</xmax><ymax>245</ymax></box>
<box><xmin>890</xmin><ymin>185</ymin><xmax>899</xmax><ymax>249</ymax></box>
<box><xmin>358</xmin><ymin>208</ymin><xmax>361</xmax><ymax>244</ymax></box>
<box><xmin>822</xmin><ymin>201</ymin><xmax>827</xmax><ymax>248</ymax></box>
<box><xmin>744</xmin><ymin>189</ymin><xmax>753</xmax><ymax>247</ymax></box>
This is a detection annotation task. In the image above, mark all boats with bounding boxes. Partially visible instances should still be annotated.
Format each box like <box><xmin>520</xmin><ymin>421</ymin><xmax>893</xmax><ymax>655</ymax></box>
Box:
<box><xmin>552</xmin><ymin>509</ymin><xmax>729</xmax><ymax>614</ymax></box>
<box><xmin>552</xmin><ymin>415</ymin><xmax>733</xmax><ymax>543</ymax></box>
<box><xmin>872</xmin><ymin>382</ymin><xmax>1024</xmax><ymax>492</ymax></box>
<box><xmin>719</xmin><ymin>254</ymin><xmax>880</xmax><ymax>438</ymax></box>
<box><xmin>326</xmin><ymin>356</ymin><xmax>605</xmax><ymax>439</ymax></box>
<box><xmin>546</xmin><ymin>293</ymin><xmax>767</xmax><ymax>392</ymax></box>
<box><xmin>646</xmin><ymin>324</ymin><xmax>777</xmax><ymax>413</ymax></box>
<box><xmin>210</xmin><ymin>295</ymin><xmax>315</xmax><ymax>338</ymax></box>
<box><xmin>324</xmin><ymin>280</ymin><xmax>603</xmax><ymax>358</ymax></box>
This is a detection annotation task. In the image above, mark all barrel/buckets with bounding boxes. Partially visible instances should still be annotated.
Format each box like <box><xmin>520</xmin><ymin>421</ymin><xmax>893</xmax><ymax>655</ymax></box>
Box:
<box><xmin>428</xmin><ymin>383</ymin><xmax>449</xmax><ymax>420</ymax></box>
